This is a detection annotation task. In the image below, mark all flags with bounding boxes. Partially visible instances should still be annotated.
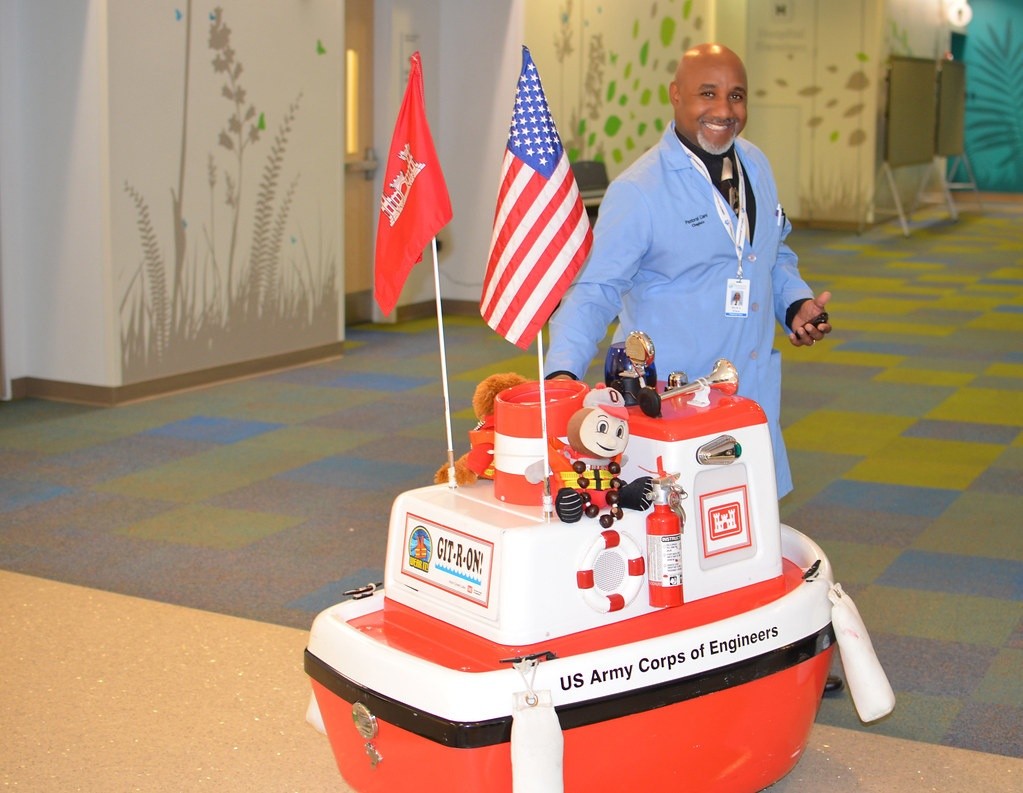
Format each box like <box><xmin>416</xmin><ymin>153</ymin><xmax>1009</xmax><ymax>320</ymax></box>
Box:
<box><xmin>373</xmin><ymin>51</ymin><xmax>453</xmax><ymax>319</ymax></box>
<box><xmin>477</xmin><ymin>48</ymin><xmax>592</xmax><ymax>350</ymax></box>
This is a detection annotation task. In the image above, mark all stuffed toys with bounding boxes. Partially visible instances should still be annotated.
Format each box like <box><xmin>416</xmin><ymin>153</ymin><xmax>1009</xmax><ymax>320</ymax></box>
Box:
<box><xmin>553</xmin><ymin>383</ymin><xmax>654</xmax><ymax>523</ymax></box>
<box><xmin>432</xmin><ymin>372</ymin><xmax>533</xmax><ymax>486</ymax></box>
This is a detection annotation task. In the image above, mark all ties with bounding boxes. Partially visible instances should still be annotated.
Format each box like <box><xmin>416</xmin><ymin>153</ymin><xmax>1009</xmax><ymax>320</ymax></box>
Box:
<box><xmin>719</xmin><ymin>156</ymin><xmax>740</xmax><ymax>221</ymax></box>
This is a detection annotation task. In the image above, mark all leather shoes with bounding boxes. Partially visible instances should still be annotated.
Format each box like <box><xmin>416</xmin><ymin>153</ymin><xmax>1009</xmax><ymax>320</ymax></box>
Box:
<box><xmin>822</xmin><ymin>674</ymin><xmax>845</xmax><ymax>698</ymax></box>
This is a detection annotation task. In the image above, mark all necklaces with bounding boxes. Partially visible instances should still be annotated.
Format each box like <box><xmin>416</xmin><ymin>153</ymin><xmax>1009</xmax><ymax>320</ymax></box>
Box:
<box><xmin>573</xmin><ymin>452</ymin><xmax>623</xmax><ymax>527</ymax></box>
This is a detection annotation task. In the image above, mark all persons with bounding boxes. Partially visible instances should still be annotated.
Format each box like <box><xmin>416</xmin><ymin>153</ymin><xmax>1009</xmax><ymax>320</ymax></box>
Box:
<box><xmin>540</xmin><ymin>42</ymin><xmax>844</xmax><ymax>697</ymax></box>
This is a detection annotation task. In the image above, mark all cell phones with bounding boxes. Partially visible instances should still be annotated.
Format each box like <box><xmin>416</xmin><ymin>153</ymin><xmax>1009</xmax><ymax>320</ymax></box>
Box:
<box><xmin>795</xmin><ymin>312</ymin><xmax>829</xmax><ymax>339</ymax></box>
